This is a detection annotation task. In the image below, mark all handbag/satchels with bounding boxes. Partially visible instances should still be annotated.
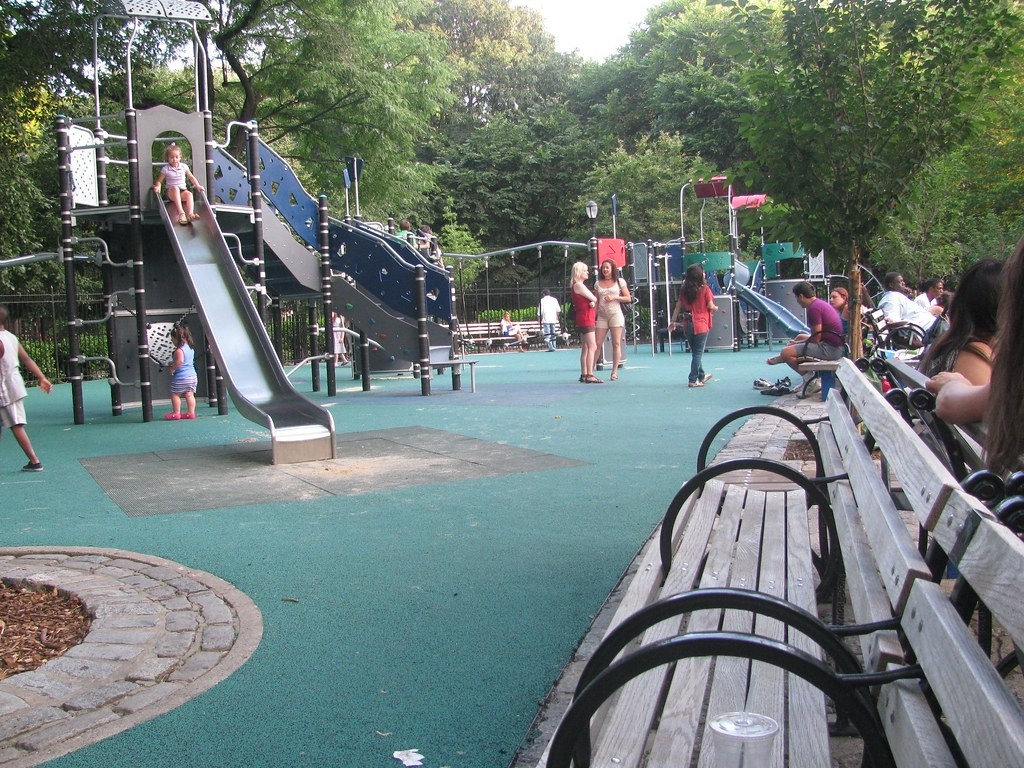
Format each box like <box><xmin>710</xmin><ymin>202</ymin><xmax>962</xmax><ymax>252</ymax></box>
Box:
<box><xmin>617</xmin><ymin>278</ymin><xmax>632</xmax><ymax>315</ymax></box>
<box><xmin>567</xmin><ymin>305</ymin><xmax>576</xmax><ymax>320</ymax></box>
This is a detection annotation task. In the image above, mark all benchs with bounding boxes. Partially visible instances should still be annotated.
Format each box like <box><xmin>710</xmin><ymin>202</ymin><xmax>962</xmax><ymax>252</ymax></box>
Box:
<box><xmin>538</xmin><ymin>308</ymin><xmax>1024</xmax><ymax>768</ymax></box>
<box><xmin>454</xmin><ymin>320</ymin><xmax>570</xmax><ymax>353</ymax></box>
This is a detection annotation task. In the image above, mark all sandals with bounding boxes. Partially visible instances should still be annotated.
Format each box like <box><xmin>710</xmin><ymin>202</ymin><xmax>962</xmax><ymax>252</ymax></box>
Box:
<box><xmin>580</xmin><ymin>374</ymin><xmax>603</xmax><ymax>383</ymax></box>
<box><xmin>611</xmin><ymin>373</ymin><xmax>618</xmax><ymax>381</ymax></box>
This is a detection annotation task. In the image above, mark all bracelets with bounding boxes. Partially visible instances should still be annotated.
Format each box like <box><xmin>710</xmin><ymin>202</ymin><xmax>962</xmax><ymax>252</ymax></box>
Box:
<box><xmin>670</xmin><ymin>319</ymin><xmax>676</xmax><ymax>322</ymax></box>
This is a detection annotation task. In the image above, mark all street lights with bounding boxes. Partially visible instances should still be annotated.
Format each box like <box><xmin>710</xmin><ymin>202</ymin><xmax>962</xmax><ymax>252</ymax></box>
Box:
<box><xmin>586</xmin><ymin>201</ymin><xmax>599</xmax><ymax>282</ymax></box>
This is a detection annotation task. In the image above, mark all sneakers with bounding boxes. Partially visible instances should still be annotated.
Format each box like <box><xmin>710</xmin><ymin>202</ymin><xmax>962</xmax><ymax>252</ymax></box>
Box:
<box><xmin>794</xmin><ymin>379</ymin><xmax>807</xmax><ymax>391</ymax></box>
<box><xmin>795</xmin><ymin>378</ymin><xmax>821</xmax><ymax>398</ymax></box>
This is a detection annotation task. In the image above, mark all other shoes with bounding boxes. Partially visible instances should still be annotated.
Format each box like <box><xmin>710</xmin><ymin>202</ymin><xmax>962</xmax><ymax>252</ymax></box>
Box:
<box><xmin>689</xmin><ymin>382</ymin><xmax>703</xmax><ymax>387</ymax></box>
<box><xmin>699</xmin><ymin>373</ymin><xmax>711</xmax><ymax>383</ymax></box>
<box><xmin>341</xmin><ymin>361</ymin><xmax>349</xmax><ymax>366</ymax></box>
<box><xmin>178</xmin><ymin>212</ymin><xmax>188</xmax><ymax>224</ymax></box>
<box><xmin>768</xmin><ymin>356</ymin><xmax>782</xmax><ymax>364</ymax></box>
<box><xmin>753</xmin><ymin>377</ymin><xmax>771</xmax><ymax>388</ymax></box>
<box><xmin>545</xmin><ymin>348</ymin><xmax>555</xmax><ymax>352</ymax></box>
<box><xmin>760</xmin><ymin>387</ymin><xmax>789</xmax><ymax>395</ymax></box>
<box><xmin>186</xmin><ymin>214</ymin><xmax>200</xmax><ymax>221</ymax></box>
<box><xmin>22</xmin><ymin>461</ymin><xmax>43</xmax><ymax>471</ymax></box>
<box><xmin>775</xmin><ymin>376</ymin><xmax>790</xmax><ymax>386</ymax></box>
<box><xmin>164</xmin><ymin>412</ymin><xmax>180</xmax><ymax>420</ymax></box>
<box><xmin>180</xmin><ymin>413</ymin><xmax>195</xmax><ymax>419</ymax></box>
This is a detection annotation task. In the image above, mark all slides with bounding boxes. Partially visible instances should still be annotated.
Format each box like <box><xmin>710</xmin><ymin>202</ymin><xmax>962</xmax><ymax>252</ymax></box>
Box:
<box><xmin>722</xmin><ymin>258</ymin><xmax>811</xmax><ymax>340</ymax></box>
<box><xmin>149</xmin><ymin>184</ymin><xmax>338</xmax><ymax>466</ymax></box>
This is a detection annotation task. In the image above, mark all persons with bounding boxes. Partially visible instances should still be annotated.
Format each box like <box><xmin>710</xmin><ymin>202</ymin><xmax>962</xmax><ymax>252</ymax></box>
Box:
<box><xmin>570</xmin><ymin>261</ymin><xmax>604</xmax><ymax>383</ymax></box>
<box><xmin>667</xmin><ymin>264</ymin><xmax>718</xmax><ymax>387</ymax></box>
<box><xmin>154</xmin><ymin>146</ymin><xmax>205</xmax><ymax>224</ymax></box>
<box><xmin>876</xmin><ymin>235</ymin><xmax>1024</xmax><ymax>476</ymax></box>
<box><xmin>331</xmin><ymin>311</ymin><xmax>349</xmax><ymax>367</ymax></box>
<box><xmin>501</xmin><ymin>311</ymin><xmax>528</xmax><ymax>353</ymax></box>
<box><xmin>0</xmin><ymin>307</ymin><xmax>52</xmax><ymax>471</ymax></box>
<box><xmin>164</xmin><ymin>326</ymin><xmax>198</xmax><ymax>420</ymax></box>
<box><xmin>537</xmin><ymin>289</ymin><xmax>562</xmax><ymax>351</ymax></box>
<box><xmin>781</xmin><ymin>281</ymin><xmax>844</xmax><ymax>399</ymax></box>
<box><xmin>767</xmin><ymin>287</ymin><xmax>849</xmax><ymax>392</ymax></box>
<box><xmin>396</xmin><ymin>219</ymin><xmax>443</xmax><ymax>268</ymax></box>
<box><xmin>593</xmin><ymin>259</ymin><xmax>631</xmax><ymax>381</ymax></box>
